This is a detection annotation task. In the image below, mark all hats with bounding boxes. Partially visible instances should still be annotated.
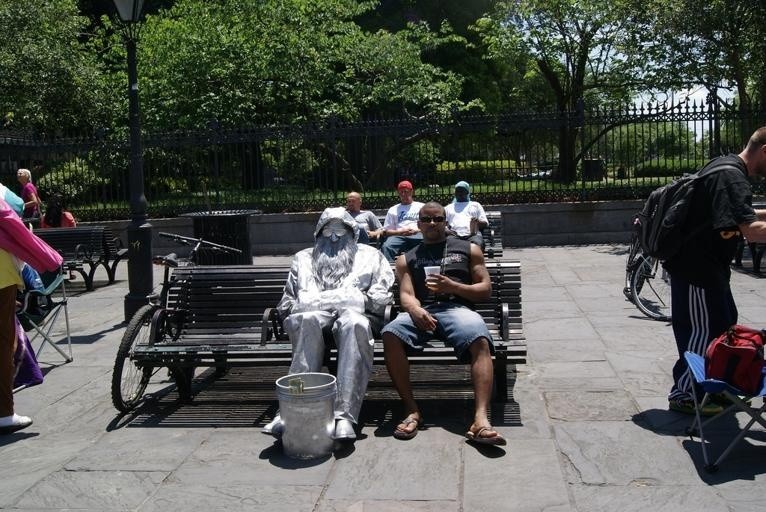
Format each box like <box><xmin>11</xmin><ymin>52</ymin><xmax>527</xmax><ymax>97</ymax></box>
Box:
<box><xmin>398</xmin><ymin>180</ymin><xmax>412</xmax><ymax>192</ymax></box>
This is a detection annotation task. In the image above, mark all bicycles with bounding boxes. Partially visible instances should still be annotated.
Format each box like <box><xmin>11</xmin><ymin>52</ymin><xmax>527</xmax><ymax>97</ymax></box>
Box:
<box><xmin>623</xmin><ymin>211</ymin><xmax>672</xmax><ymax>322</ymax></box>
<box><xmin>112</xmin><ymin>232</ymin><xmax>243</xmax><ymax>416</ymax></box>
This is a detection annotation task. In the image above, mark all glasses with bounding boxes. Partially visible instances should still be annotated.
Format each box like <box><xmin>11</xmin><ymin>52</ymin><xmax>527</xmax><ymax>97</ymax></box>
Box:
<box><xmin>419</xmin><ymin>214</ymin><xmax>446</xmax><ymax>224</ymax></box>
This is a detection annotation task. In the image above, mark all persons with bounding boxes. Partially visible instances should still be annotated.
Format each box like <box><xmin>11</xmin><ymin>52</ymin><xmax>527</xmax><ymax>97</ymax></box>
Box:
<box><xmin>511</xmin><ymin>170</ymin><xmax>546</xmax><ymax>181</ymax></box>
<box><xmin>440</xmin><ymin>180</ymin><xmax>490</xmax><ymax>252</ymax></box>
<box><xmin>618</xmin><ymin>166</ymin><xmax>626</xmax><ymax>179</ymax></box>
<box><xmin>380</xmin><ymin>201</ymin><xmax>506</xmax><ymax>446</ymax></box>
<box><xmin>345</xmin><ymin>191</ymin><xmax>380</xmax><ymax>246</ymax></box>
<box><xmin>382</xmin><ymin>181</ymin><xmax>428</xmax><ymax>263</ymax></box>
<box><xmin>260</xmin><ymin>205</ymin><xmax>395</xmax><ymax>439</ymax></box>
<box><xmin>661</xmin><ymin>127</ymin><xmax>766</xmax><ymax>417</ymax></box>
<box><xmin>365</xmin><ymin>219</ymin><xmax>457</xmax><ymax>240</ymax></box>
<box><xmin>0</xmin><ymin>168</ymin><xmax>75</xmax><ymax>437</ymax></box>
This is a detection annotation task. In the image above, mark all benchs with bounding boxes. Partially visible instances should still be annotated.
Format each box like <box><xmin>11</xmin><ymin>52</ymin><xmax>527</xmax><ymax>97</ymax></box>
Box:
<box><xmin>367</xmin><ymin>215</ymin><xmax>385</xmax><ymax>250</ymax></box>
<box><xmin>732</xmin><ymin>200</ymin><xmax>766</xmax><ymax>278</ymax></box>
<box><xmin>127</xmin><ymin>265</ymin><xmax>527</xmax><ymax>406</ymax></box>
<box><xmin>31</xmin><ymin>224</ymin><xmax>106</xmax><ymax>294</ymax></box>
<box><xmin>58</xmin><ymin>228</ymin><xmax>135</xmax><ymax>284</ymax></box>
<box><xmin>480</xmin><ymin>211</ymin><xmax>504</xmax><ymax>258</ymax></box>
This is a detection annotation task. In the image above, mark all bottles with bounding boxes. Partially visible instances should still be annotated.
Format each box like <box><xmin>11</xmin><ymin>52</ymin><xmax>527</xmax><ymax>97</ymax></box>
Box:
<box><xmin>470</xmin><ymin>220</ymin><xmax>478</xmax><ymax>235</ymax></box>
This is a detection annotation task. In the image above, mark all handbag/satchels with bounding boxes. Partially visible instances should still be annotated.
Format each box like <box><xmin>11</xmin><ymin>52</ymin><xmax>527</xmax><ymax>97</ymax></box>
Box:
<box><xmin>704</xmin><ymin>319</ymin><xmax>765</xmax><ymax>392</ymax></box>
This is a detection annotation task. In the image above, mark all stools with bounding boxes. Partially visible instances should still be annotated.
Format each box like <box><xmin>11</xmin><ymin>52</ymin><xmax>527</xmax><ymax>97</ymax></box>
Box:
<box><xmin>683</xmin><ymin>348</ymin><xmax>765</xmax><ymax>475</ymax></box>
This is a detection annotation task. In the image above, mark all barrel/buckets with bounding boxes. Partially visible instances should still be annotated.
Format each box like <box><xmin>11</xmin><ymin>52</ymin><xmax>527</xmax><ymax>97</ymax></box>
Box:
<box><xmin>582</xmin><ymin>157</ymin><xmax>604</xmax><ymax>182</ymax></box>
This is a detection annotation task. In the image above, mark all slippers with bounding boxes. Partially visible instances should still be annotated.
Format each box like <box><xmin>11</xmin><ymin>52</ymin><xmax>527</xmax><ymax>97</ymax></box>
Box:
<box><xmin>391</xmin><ymin>415</ymin><xmax>426</xmax><ymax>439</ymax></box>
<box><xmin>464</xmin><ymin>425</ymin><xmax>508</xmax><ymax>450</ymax></box>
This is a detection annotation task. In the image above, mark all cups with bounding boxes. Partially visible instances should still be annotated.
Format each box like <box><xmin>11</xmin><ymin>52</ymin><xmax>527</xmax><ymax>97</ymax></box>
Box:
<box><xmin>423</xmin><ymin>265</ymin><xmax>441</xmax><ymax>288</ymax></box>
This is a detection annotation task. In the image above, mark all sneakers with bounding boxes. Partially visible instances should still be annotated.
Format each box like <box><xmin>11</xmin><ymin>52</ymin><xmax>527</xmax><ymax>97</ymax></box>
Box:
<box><xmin>668</xmin><ymin>395</ymin><xmax>752</xmax><ymax>415</ymax></box>
<box><xmin>0</xmin><ymin>414</ymin><xmax>32</xmax><ymax>428</ymax></box>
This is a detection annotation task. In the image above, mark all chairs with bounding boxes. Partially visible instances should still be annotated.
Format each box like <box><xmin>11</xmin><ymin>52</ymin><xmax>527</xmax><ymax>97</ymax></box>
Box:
<box><xmin>14</xmin><ymin>273</ymin><xmax>75</xmax><ymax>369</ymax></box>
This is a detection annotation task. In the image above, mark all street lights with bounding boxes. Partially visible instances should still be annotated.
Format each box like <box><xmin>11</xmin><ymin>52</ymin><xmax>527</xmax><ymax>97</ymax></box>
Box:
<box><xmin>74</xmin><ymin>1</ymin><xmax>176</xmax><ymax>322</ymax></box>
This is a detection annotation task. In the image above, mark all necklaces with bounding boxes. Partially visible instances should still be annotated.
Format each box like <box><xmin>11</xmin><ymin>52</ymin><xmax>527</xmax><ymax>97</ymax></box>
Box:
<box><xmin>422</xmin><ymin>243</ymin><xmax>447</xmax><ymax>296</ymax></box>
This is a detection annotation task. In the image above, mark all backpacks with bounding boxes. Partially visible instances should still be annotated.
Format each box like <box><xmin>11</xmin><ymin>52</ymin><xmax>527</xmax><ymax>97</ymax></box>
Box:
<box><xmin>641</xmin><ymin>158</ymin><xmax>745</xmax><ymax>261</ymax></box>
